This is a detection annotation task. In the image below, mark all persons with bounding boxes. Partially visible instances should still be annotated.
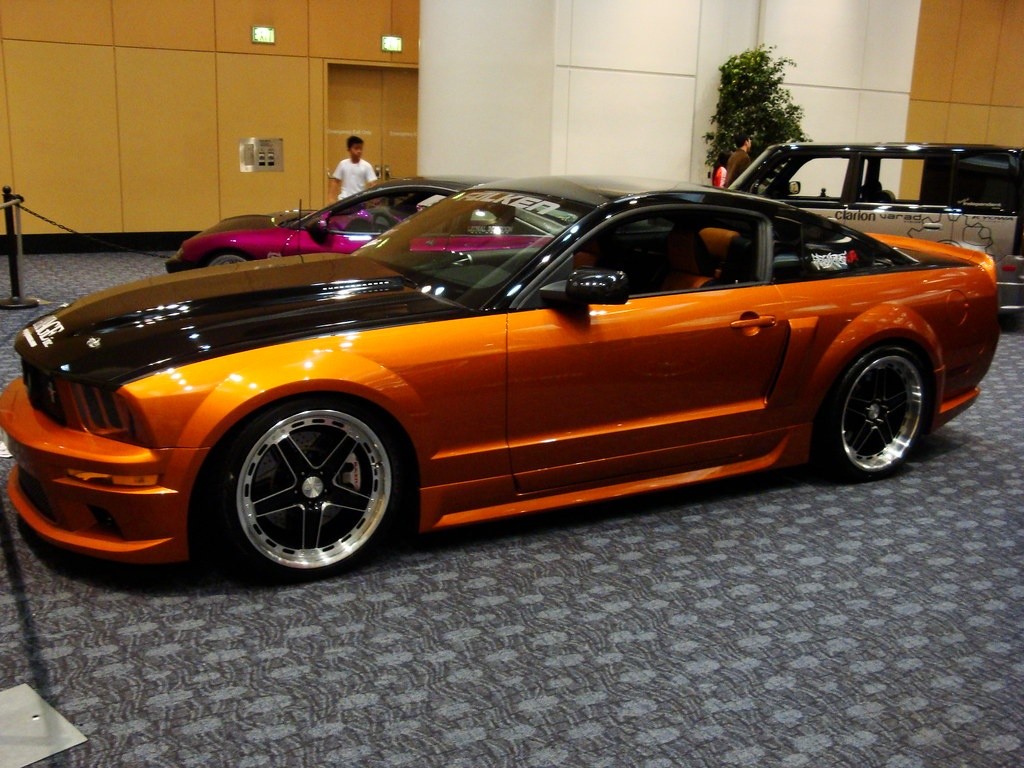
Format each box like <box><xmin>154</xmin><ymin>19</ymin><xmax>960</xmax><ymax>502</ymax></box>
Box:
<box><xmin>712</xmin><ymin>132</ymin><xmax>752</xmax><ymax>189</ymax></box>
<box><xmin>328</xmin><ymin>135</ymin><xmax>378</xmax><ymax>206</ymax></box>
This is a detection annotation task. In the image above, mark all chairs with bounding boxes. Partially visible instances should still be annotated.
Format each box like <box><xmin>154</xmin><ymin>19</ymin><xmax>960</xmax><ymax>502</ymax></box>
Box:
<box><xmin>573</xmin><ymin>239</ymin><xmax>599</xmax><ymax>268</ymax></box>
<box><xmin>658</xmin><ymin>228</ymin><xmax>740</xmax><ymax>290</ymax></box>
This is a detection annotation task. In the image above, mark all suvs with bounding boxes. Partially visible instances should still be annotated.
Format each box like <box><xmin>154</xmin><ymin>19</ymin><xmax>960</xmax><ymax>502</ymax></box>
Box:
<box><xmin>722</xmin><ymin>139</ymin><xmax>1024</xmax><ymax>312</ymax></box>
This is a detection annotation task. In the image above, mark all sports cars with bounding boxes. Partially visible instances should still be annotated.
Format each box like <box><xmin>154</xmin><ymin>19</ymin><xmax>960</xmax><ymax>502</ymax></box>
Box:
<box><xmin>0</xmin><ymin>179</ymin><xmax>1001</xmax><ymax>580</ymax></box>
<box><xmin>163</xmin><ymin>179</ymin><xmax>566</xmax><ymax>274</ymax></box>
